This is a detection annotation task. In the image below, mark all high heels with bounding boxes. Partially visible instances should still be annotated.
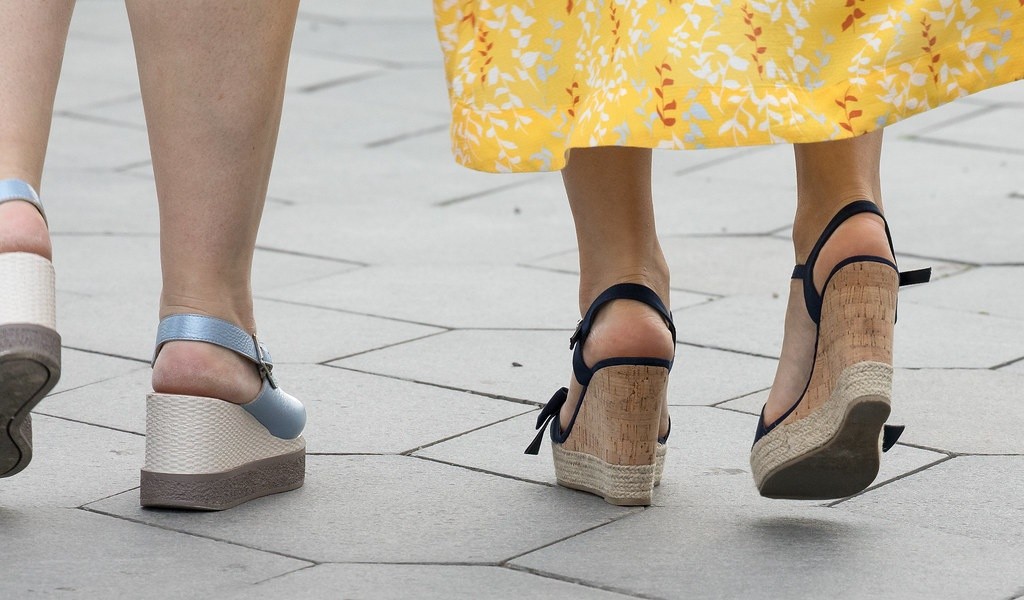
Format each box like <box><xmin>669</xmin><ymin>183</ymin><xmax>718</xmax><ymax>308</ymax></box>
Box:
<box><xmin>749</xmin><ymin>200</ymin><xmax>932</xmax><ymax>498</ymax></box>
<box><xmin>141</xmin><ymin>314</ymin><xmax>306</xmax><ymax>509</ymax></box>
<box><xmin>0</xmin><ymin>179</ymin><xmax>61</xmax><ymax>478</ymax></box>
<box><xmin>524</xmin><ymin>283</ymin><xmax>676</xmax><ymax>507</ymax></box>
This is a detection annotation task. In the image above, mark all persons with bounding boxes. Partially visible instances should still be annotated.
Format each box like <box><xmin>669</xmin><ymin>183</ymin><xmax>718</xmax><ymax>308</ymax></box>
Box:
<box><xmin>434</xmin><ymin>1</ymin><xmax>1024</xmax><ymax>507</ymax></box>
<box><xmin>0</xmin><ymin>0</ymin><xmax>306</xmax><ymax>513</ymax></box>
